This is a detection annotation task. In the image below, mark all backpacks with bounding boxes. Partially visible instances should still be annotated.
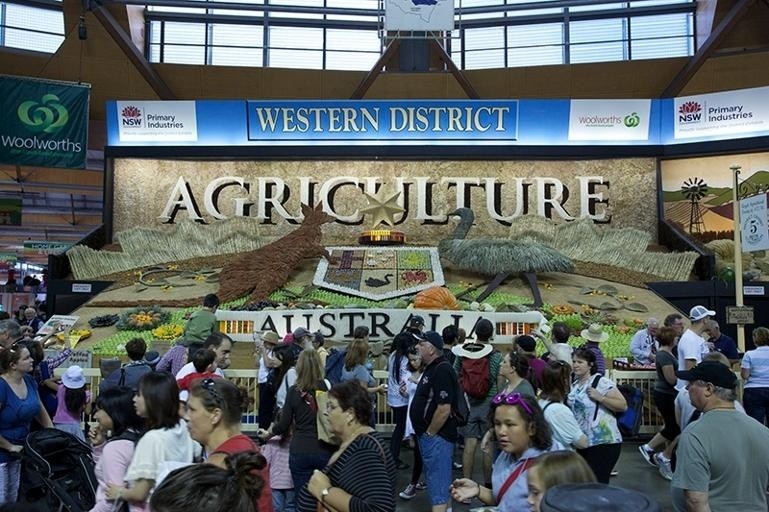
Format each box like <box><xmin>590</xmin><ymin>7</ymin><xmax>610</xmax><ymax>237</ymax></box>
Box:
<box><xmin>591</xmin><ymin>374</ymin><xmax>644</xmax><ymax>436</ymax></box>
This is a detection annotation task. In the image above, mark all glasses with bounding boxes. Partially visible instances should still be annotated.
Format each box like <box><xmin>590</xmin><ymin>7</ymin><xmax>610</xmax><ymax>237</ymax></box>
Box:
<box><xmin>200</xmin><ymin>378</ymin><xmax>221</xmax><ymax>405</ymax></box>
<box><xmin>492</xmin><ymin>393</ymin><xmax>533</xmax><ymax>416</ymax></box>
<box><xmin>9</xmin><ymin>343</ymin><xmax>18</xmax><ymax>360</ymax></box>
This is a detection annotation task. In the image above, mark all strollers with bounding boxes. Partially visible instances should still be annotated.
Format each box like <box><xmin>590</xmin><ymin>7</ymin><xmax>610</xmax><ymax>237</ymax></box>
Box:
<box><xmin>8</xmin><ymin>427</ymin><xmax>98</xmax><ymax>511</ymax></box>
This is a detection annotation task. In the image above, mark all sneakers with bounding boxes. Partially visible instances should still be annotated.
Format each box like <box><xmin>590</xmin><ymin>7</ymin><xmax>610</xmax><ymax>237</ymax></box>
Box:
<box><xmin>638</xmin><ymin>445</ymin><xmax>658</xmax><ymax>469</ymax></box>
<box><xmin>399</xmin><ymin>484</ymin><xmax>416</xmax><ymax>499</ymax></box>
<box><xmin>397</xmin><ymin>460</ymin><xmax>409</xmax><ymax>469</ymax></box>
<box><xmin>416</xmin><ymin>481</ymin><xmax>426</xmax><ymax>489</ymax></box>
<box><xmin>653</xmin><ymin>453</ymin><xmax>673</xmax><ymax>480</ymax></box>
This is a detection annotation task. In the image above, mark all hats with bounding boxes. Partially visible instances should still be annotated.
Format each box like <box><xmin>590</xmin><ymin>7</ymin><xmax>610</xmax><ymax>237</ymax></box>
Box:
<box><xmin>675</xmin><ymin>360</ymin><xmax>737</xmax><ymax>387</ymax></box>
<box><xmin>143</xmin><ymin>351</ymin><xmax>161</xmax><ymax>365</ymax></box>
<box><xmin>690</xmin><ymin>305</ymin><xmax>716</xmax><ymax>322</ymax></box>
<box><xmin>63</xmin><ymin>365</ymin><xmax>86</xmax><ymax>387</ymax></box>
<box><xmin>259</xmin><ymin>315</ymin><xmax>534</xmax><ymax>388</ymax></box>
<box><xmin>581</xmin><ymin>323</ymin><xmax>609</xmax><ymax>343</ymax></box>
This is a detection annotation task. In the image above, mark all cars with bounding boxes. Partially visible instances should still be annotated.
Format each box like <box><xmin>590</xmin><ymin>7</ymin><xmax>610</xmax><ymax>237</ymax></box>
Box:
<box><xmin>0</xmin><ymin>259</ymin><xmax>46</xmax><ymax>293</ymax></box>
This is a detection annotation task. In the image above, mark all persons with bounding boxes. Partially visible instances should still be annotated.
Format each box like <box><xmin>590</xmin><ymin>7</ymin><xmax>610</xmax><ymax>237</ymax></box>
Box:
<box><xmin>0</xmin><ymin>263</ymin><xmax>46</xmax><ymax>302</ymax></box>
<box><xmin>1</xmin><ymin>294</ymin><xmax>769</xmax><ymax>512</ymax></box>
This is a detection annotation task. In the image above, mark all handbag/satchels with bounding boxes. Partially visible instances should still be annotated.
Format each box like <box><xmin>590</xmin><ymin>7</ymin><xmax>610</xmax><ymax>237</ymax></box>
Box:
<box><xmin>436</xmin><ymin>362</ymin><xmax>470</xmax><ymax>426</ymax></box>
<box><xmin>316</xmin><ymin>391</ymin><xmax>341</xmax><ymax>445</ymax></box>
